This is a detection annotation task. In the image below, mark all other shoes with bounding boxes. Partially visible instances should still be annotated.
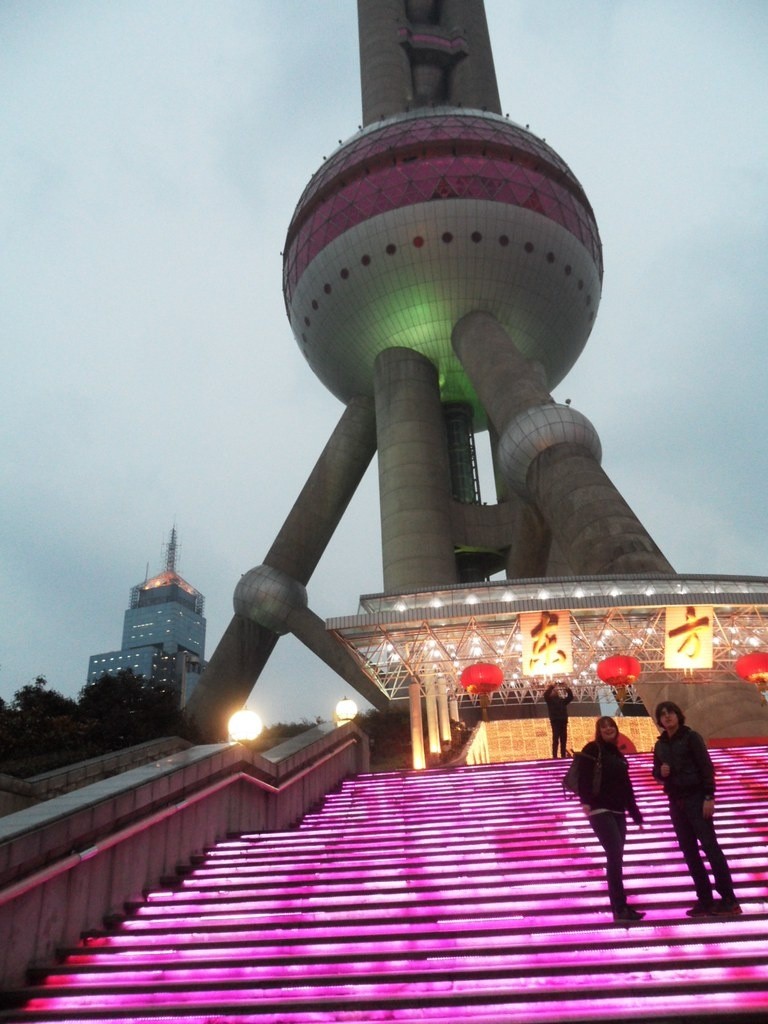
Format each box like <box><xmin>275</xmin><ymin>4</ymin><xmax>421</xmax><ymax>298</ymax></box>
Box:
<box><xmin>613</xmin><ymin>910</ymin><xmax>646</xmax><ymax>920</ymax></box>
<box><xmin>710</xmin><ymin>903</ymin><xmax>743</xmax><ymax>915</ymax></box>
<box><xmin>686</xmin><ymin>904</ymin><xmax>710</xmax><ymax>917</ymax></box>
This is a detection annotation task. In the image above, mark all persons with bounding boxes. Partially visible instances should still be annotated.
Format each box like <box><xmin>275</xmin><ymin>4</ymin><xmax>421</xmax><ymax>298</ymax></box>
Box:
<box><xmin>561</xmin><ymin>715</ymin><xmax>648</xmax><ymax>920</ymax></box>
<box><xmin>543</xmin><ymin>681</ymin><xmax>575</xmax><ymax>758</ymax></box>
<box><xmin>651</xmin><ymin>700</ymin><xmax>745</xmax><ymax>916</ymax></box>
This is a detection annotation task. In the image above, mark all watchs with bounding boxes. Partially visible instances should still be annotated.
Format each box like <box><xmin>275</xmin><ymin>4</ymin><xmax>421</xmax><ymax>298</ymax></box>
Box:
<box><xmin>704</xmin><ymin>794</ymin><xmax>714</xmax><ymax>801</ymax></box>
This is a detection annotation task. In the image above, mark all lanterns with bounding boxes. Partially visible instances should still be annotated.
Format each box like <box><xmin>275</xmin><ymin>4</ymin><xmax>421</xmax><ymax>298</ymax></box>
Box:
<box><xmin>597</xmin><ymin>653</ymin><xmax>641</xmax><ymax>707</ymax></box>
<box><xmin>735</xmin><ymin>649</ymin><xmax>768</xmax><ymax>707</ymax></box>
<box><xmin>461</xmin><ymin>660</ymin><xmax>503</xmax><ymax>723</ymax></box>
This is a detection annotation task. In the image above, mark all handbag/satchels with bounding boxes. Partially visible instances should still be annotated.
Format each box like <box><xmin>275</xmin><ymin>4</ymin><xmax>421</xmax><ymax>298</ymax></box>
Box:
<box><xmin>562</xmin><ymin>742</ymin><xmax>602</xmax><ymax>801</ymax></box>
